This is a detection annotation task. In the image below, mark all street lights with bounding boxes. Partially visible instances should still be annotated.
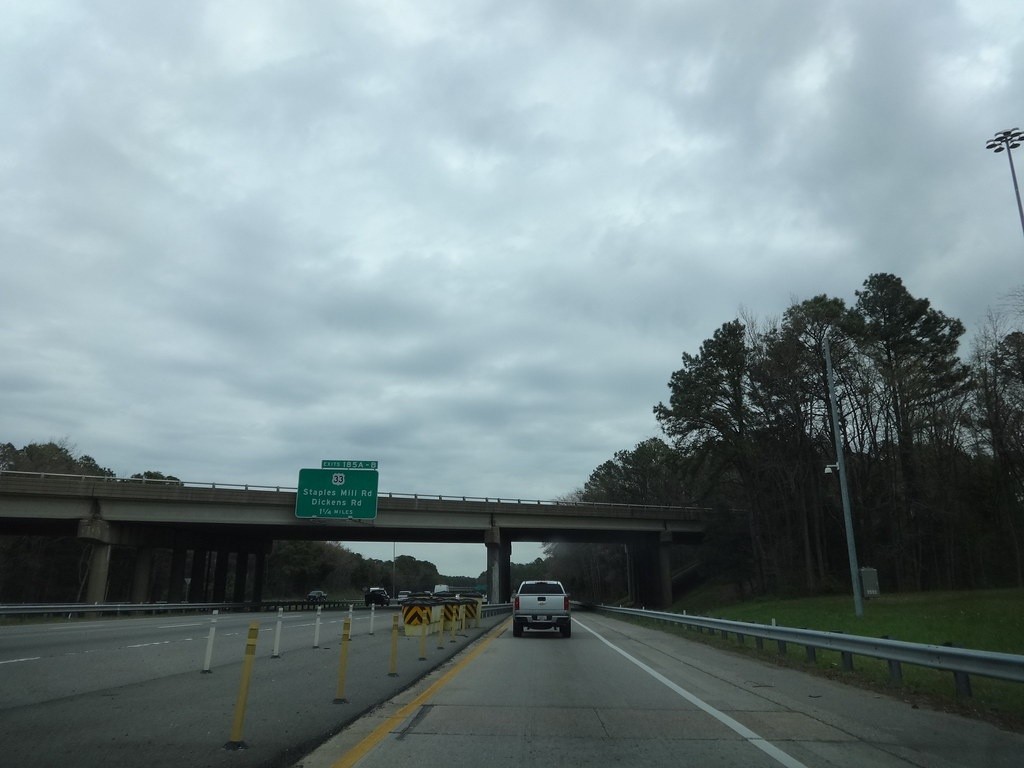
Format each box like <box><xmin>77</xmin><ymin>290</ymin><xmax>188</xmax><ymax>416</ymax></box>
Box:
<box><xmin>987</xmin><ymin>126</ymin><xmax>1023</xmax><ymax>235</ymax></box>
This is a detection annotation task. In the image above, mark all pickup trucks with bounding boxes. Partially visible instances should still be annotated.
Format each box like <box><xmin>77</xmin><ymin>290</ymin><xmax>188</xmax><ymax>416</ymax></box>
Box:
<box><xmin>510</xmin><ymin>580</ymin><xmax>572</xmax><ymax>638</ymax></box>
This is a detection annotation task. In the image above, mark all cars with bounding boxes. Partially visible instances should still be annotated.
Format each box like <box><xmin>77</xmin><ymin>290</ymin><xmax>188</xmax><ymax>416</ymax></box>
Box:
<box><xmin>306</xmin><ymin>590</ymin><xmax>327</xmax><ymax>601</ymax></box>
<box><xmin>398</xmin><ymin>584</ymin><xmax>487</xmax><ymax>605</ymax></box>
<box><xmin>365</xmin><ymin>590</ymin><xmax>388</xmax><ymax>607</ymax></box>
